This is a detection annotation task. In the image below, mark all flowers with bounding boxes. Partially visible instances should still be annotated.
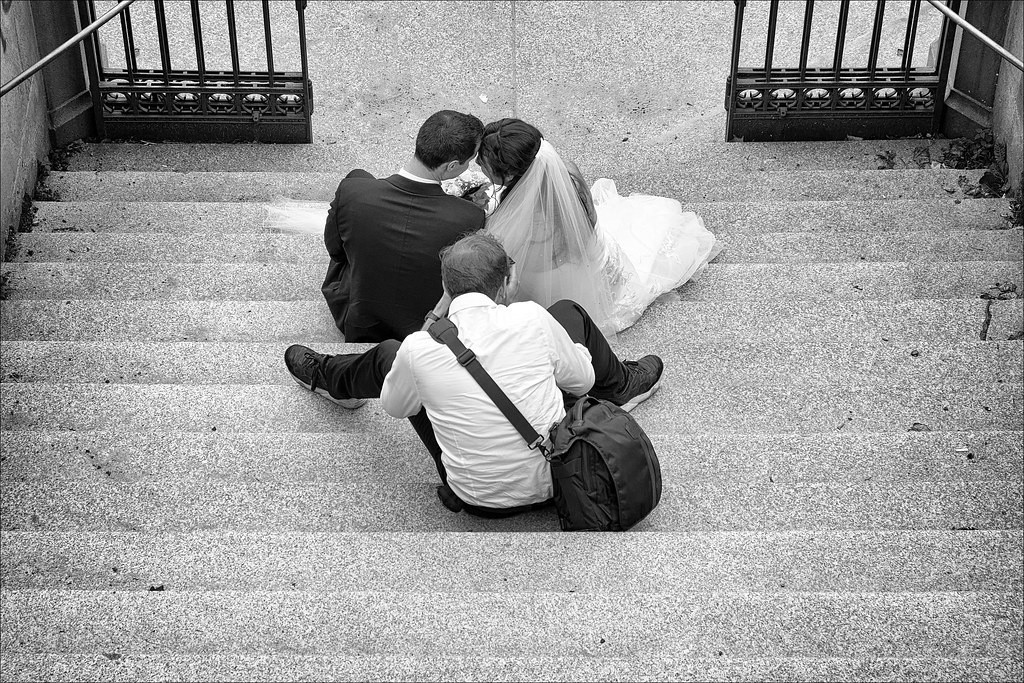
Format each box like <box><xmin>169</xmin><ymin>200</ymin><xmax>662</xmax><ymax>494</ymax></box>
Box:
<box><xmin>443</xmin><ymin>169</ymin><xmax>493</xmax><ymax>211</ymax></box>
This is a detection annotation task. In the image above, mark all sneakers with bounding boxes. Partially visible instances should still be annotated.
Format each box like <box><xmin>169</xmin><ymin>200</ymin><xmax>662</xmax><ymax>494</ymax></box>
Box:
<box><xmin>284</xmin><ymin>344</ymin><xmax>367</xmax><ymax>409</ymax></box>
<box><xmin>606</xmin><ymin>355</ymin><xmax>664</xmax><ymax>413</ymax></box>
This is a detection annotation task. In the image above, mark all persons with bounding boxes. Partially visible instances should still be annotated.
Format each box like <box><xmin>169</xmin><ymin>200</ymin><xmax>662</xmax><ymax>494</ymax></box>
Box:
<box><xmin>321</xmin><ymin>110</ymin><xmax>486</xmax><ymax>341</ymax></box>
<box><xmin>285</xmin><ymin>230</ymin><xmax>665</xmax><ymax>520</ymax></box>
<box><xmin>475</xmin><ymin>118</ymin><xmax>723</xmax><ymax>350</ymax></box>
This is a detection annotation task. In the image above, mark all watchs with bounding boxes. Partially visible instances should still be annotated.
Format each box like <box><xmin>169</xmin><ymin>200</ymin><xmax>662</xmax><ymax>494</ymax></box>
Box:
<box><xmin>424</xmin><ymin>309</ymin><xmax>439</xmax><ymax>323</ymax></box>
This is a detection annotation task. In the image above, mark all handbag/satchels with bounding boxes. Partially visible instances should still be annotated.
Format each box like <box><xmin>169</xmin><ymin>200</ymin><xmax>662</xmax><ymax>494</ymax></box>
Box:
<box><xmin>545</xmin><ymin>396</ymin><xmax>662</xmax><ymax>531</ymax></box>
